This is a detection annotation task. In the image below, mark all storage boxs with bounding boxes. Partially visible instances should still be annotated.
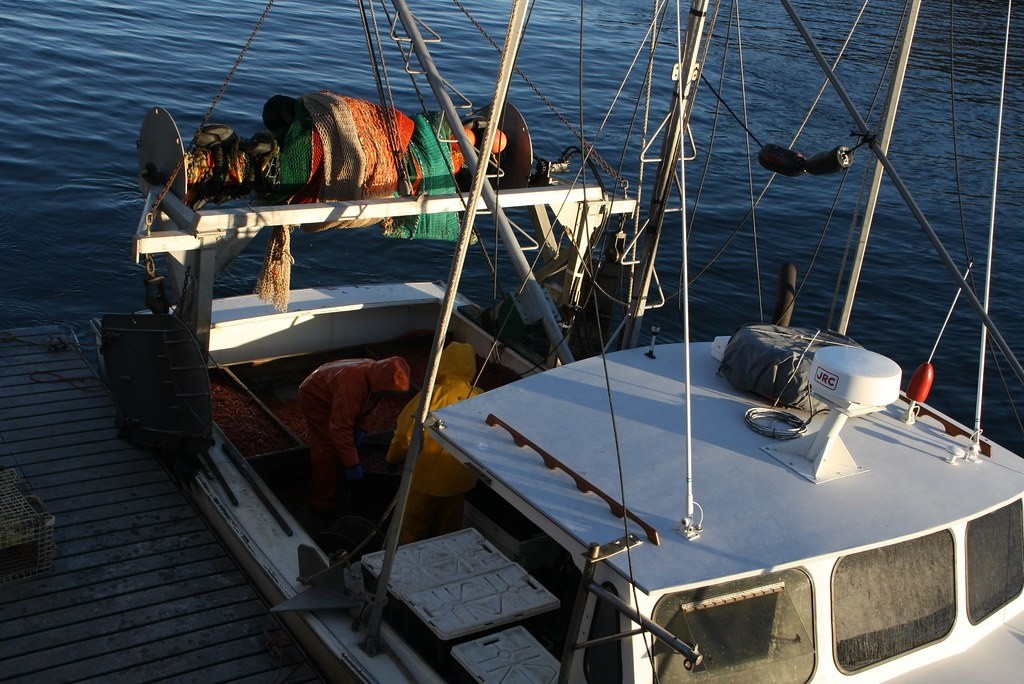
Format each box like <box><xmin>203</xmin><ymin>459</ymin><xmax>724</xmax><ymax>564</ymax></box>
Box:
<box><xmin>397</xmin><ymin>563</ymin><xmax>561</xmax><ymax>680</ymax></box>
<box><xmin>363</xmin><ymin>528</ymin><xmax>514</xmax><ymax>629</ymax></box>
<box><xmin>449</xmin><ymin>625</ymin><xmax>561</xmax><ymax>684</ymax></box>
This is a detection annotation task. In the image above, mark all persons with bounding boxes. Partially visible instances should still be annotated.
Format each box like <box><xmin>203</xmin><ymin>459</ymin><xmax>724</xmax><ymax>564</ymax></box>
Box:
<box><xmin>384</xmin><ymin>341</ymin><xmax>487</xmax><ymax>546</ymax></box>
<box><xmin>297</xmin><ymin>355</ymin><xmax>412</xmax><ymax>487</ymax></box>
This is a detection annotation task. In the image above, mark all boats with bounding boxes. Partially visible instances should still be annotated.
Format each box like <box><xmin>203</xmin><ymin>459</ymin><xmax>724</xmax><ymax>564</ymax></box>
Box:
<box><xmin>87</xmin><ymin>1</ymin><xmax>1024</xmax><ymax>684</ymax></box>
